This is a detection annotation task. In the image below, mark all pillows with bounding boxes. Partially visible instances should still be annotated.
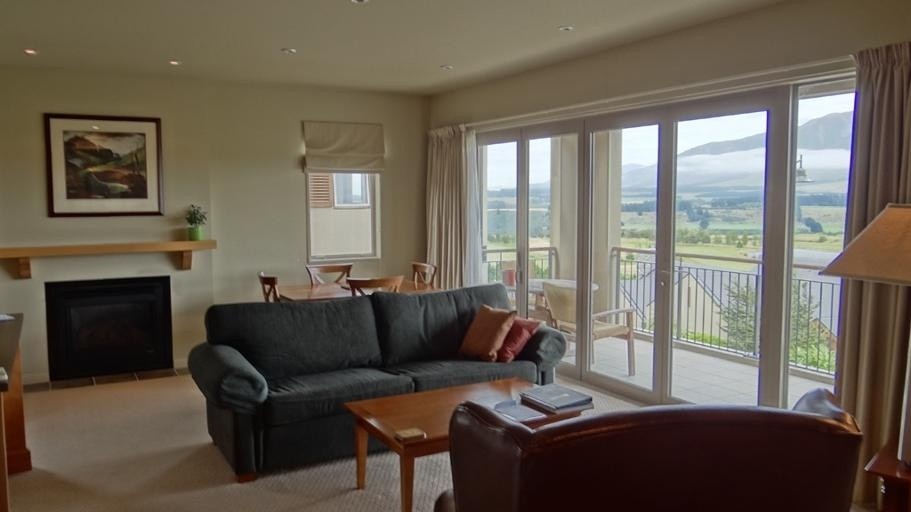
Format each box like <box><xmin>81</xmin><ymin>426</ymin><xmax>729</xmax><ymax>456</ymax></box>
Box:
<box><xmin>497</xmin><ymin>313</ymin><xmax>543</xmax><ymax>362</ymax></box>
<box><xmin>459</xmin><ymin>303</ymin><xmax>520</xmax><ymax>362</ymax></box>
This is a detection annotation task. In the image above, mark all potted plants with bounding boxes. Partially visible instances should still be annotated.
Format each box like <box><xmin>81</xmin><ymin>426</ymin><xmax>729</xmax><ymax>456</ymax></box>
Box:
<box><xmin>184</xmin><ymin>204</ymin><xmax>211</xmax><ymax>241</ymax></box>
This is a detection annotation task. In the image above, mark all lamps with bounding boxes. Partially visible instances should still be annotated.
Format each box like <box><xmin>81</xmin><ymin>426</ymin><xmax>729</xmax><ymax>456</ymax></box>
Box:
<box><xmin>816</xmin><ymin>200</ymin><xmax>911</xmax><ymax>471</ymax></box>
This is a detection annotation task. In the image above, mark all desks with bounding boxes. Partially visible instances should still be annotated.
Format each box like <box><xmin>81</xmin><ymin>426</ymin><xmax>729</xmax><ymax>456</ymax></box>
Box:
<box><xmin>864</xmin><ymin>433</ymin><xmax>911</xmax><ymax>512</ymax></box>
<box><xmin>267</xmin><ymin>279</ymin><xmax>442</xmax><ymax>303</ymax></box>
<box><xmin>502</xmin><ymin>277</ymin><xmax>602</xmax><ymax>327</ymax></box>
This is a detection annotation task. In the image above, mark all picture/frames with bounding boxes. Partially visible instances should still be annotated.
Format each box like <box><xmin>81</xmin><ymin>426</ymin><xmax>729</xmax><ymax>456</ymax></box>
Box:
<box><xmin>44</xmin><ymin>111</ymin><xmax>165</xmax><ymax>219</ymax></box>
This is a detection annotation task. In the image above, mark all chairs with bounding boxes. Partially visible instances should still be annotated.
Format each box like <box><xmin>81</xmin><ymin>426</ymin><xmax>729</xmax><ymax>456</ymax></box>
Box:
<box><xmin>347</xmin><ymin>274</ymin><xmax>407</xmax><ymax>296</ymax></box>
<box><xmin>306</xmin><ymin>262</ymin><xmax>355</xmax><ymax>284</ymax></box>
<box><xmin>542</xmin><ymin>280</ymin><xmax>637</xmax><ymax>379</ymax></box>
<box><xmin>257</xmin><ymin>272</ymin><xmax>284</xmax><ymax>302</ymax></box>
<box><xmin>412</xmin><ymin>262</ymin><xmax>439</xmax><ymax>287</ymax></box>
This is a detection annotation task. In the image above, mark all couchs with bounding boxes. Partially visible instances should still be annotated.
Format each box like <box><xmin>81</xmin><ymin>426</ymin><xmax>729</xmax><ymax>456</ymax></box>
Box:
<box><xmin>187</xmin><ymin>282</ymin><xmax>567</xmax><ymax>483</ymax></box>
<box><xmin>429</xmin><ymin>385</ymin><xmax>866</xmax><ymax>511</ymax></box>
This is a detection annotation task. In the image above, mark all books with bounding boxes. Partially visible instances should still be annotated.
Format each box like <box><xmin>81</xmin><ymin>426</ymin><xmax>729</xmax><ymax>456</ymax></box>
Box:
<box><xmin>495</xmin><ymin>383</ymin><xmax>595</xmax><ymax>426</ymax></box>
